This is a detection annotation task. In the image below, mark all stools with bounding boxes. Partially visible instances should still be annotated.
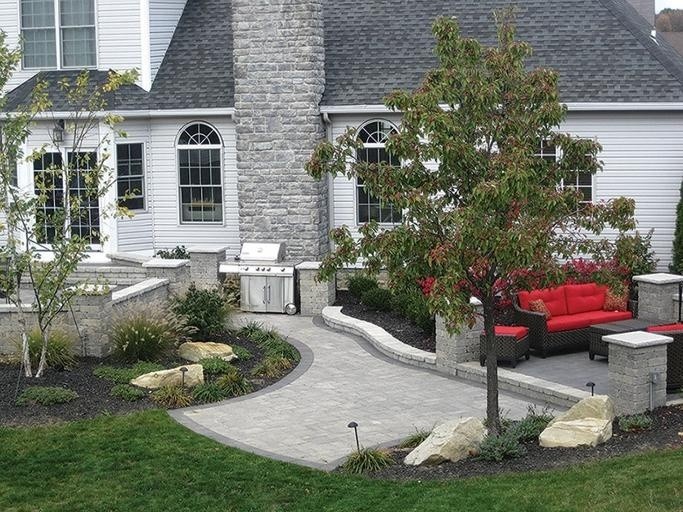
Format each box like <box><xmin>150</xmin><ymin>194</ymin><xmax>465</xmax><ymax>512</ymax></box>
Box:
<box><xmin>479</xmin><ymin>325</ymin><xmax>530</xmax><ymax>369</ymax></box>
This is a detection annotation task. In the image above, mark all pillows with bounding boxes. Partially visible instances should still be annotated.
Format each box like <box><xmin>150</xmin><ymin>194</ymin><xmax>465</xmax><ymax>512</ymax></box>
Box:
<box><xmin>528</xmin><ymin>298</ymin><xmax>552</xmax><ymax>321</ymax></box>
<box><xmin>602</xmin><ymin>281</ymin><xmax>628</xmax><ymax>312</ymax></box>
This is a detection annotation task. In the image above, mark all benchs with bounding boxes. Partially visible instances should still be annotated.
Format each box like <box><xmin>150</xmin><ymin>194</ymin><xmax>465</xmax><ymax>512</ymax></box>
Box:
<box><xmin>586</xmin><ymin>319</ymin><xmax>658</xmax><ymax>361</ymax></box>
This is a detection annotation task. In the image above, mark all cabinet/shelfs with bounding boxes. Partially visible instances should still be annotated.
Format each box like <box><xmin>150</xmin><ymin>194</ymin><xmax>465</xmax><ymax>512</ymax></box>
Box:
<box><xmin>241</xmin><ymin>277</ymin><xmax>285</xmax><ymax>315</ymax></box>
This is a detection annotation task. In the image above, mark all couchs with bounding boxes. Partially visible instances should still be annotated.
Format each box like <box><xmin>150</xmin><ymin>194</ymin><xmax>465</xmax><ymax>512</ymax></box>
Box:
<box><xmin>644</xmin><ymin>324</ymin><xmax>683</xmax><ymax>394</ymax></box>
<box><xmin>511</xmin><ymin>281</ymin><xmax>632</xmax><ymax>358</ymax></box>
